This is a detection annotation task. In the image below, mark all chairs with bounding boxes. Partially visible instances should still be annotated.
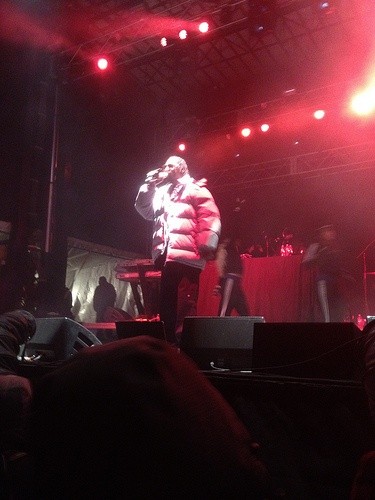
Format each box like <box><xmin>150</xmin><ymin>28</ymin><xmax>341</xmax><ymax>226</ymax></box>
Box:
<box><xmin>25</xmin><ymin>334</ymin><xmax>281</xmax><ymax>499</ymax></box>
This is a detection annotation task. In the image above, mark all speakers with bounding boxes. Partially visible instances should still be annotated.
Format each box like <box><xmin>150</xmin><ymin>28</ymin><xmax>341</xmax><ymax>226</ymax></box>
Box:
<box><xmin>17</xmin><ymin>316</ymin><xmax>363</xmax><ymax>382</ymax></box>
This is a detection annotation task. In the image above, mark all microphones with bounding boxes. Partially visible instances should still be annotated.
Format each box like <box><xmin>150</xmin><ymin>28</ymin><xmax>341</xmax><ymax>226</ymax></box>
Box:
<box><xmin>145</xmin><ymin>168</ymin><xmax>162</xmax><ymax>182</ymax></box>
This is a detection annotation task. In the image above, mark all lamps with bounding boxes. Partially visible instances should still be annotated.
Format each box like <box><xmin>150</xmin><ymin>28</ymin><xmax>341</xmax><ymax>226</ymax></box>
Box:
<box><xmin>22</xmin><ymin>316</ymin><xmax>103</xmax><ymax>363</ymax></box>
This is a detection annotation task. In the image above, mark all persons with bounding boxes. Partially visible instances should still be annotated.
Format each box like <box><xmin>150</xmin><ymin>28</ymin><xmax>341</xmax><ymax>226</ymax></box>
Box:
<box><xmin>0</xmin><ymin>296</ymin><xmax>278</xmax><ymax>500</ymax></box>
<box><xmin>300</xmin><ymin>224</ymin><xmax>355</xmax><ymax>323</ymax></box>
<box><xmin>93</xmin><ymin>276</ymin><xmax>117</xmax><ymax>323</ymax></box>
<box><xmin>135</xmin><ymin>156</ymin><xmax>221</xmax><ymax>348</ymax></box>
<box><xmin>212</xmin><ymin>227</ymin><xmax>252</xmax><ymax>318</ymax></box>
